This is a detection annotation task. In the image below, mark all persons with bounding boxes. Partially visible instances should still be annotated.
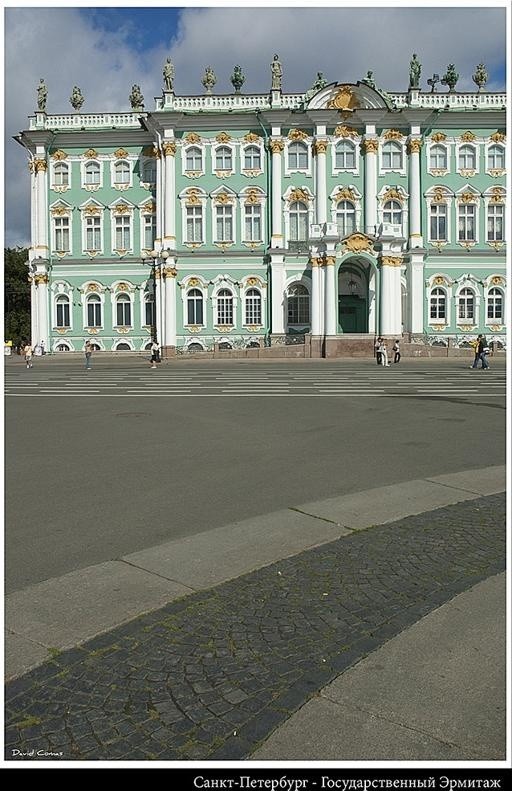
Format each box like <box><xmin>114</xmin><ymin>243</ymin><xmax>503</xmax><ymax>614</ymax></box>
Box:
<box><xmin>84</xmin><ymin>340</ymin><xmax>93</xmax><ymax>370</ymax></box>
<box><xmin>379</xmin><ymin>338</ymin><xmax>391</xmax><ymax>367</ymax></box>
<box><xmin>163</xmin><ymin>59</ymin><xmax>175</xmax><ymax>90</ymax></box>
<box><xmin>24</xmin><ymin>342</ymin><xmax>34</xmax><ymax>369</ymax></box>
<box><xmin>20</xmin><ymin>341</ymin><xmax>27</xmax><ymax>359</ymax></box>
<box><xmin>155</xmin><ymin>343</ymin><xmax>161</xmax><ymax>363</ymax></box>
<box><xmin>37</xmin><ymin>79</ymin><xmax>48</xmax><ymax>110</ymax></box>
<box><xmin>149</xmin><ymin>340</ymin><xmax>159</xmax><ymax>369</ymax></box>
<box><xmin>409</xmin><ymin>54</ymin><xmax>422</xmax><ymax>87</ymax></box>
<box><xmin>373</xmin><ymin>338</ymin><xmax>382</xmax><ymax>365</ymax></box>
<box><xmin>41</xmin><ymin>340</ymin><xmax>46</xmax><ymax>357</ymax></box>
<box><xmin>393</xmin><ymin>340</ymin><xmax>402</xmax><ymax>364</ymax></box>
<box><xmin>477</xmin><ymin>334</ymin><xmax>490</xmax><ymax>371</ymax></box>
<box><xmin>269</xmin><ymin>54</ymin><xmax>283</xmax><ymax>89</ymax></box>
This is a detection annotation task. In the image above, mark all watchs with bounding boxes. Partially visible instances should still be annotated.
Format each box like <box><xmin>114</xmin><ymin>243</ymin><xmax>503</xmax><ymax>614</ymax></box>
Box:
<box><xmin>468</xmin><ymin>338</ymin><xmax>486</xmax><ymax>370</ymax></box>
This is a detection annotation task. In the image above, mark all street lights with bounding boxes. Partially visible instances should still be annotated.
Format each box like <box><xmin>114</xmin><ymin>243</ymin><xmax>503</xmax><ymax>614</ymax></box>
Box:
<box><xmin>140</xmin><ymin>248</ymin><xmax>171</xmax><ymax>364</ymax></box>
